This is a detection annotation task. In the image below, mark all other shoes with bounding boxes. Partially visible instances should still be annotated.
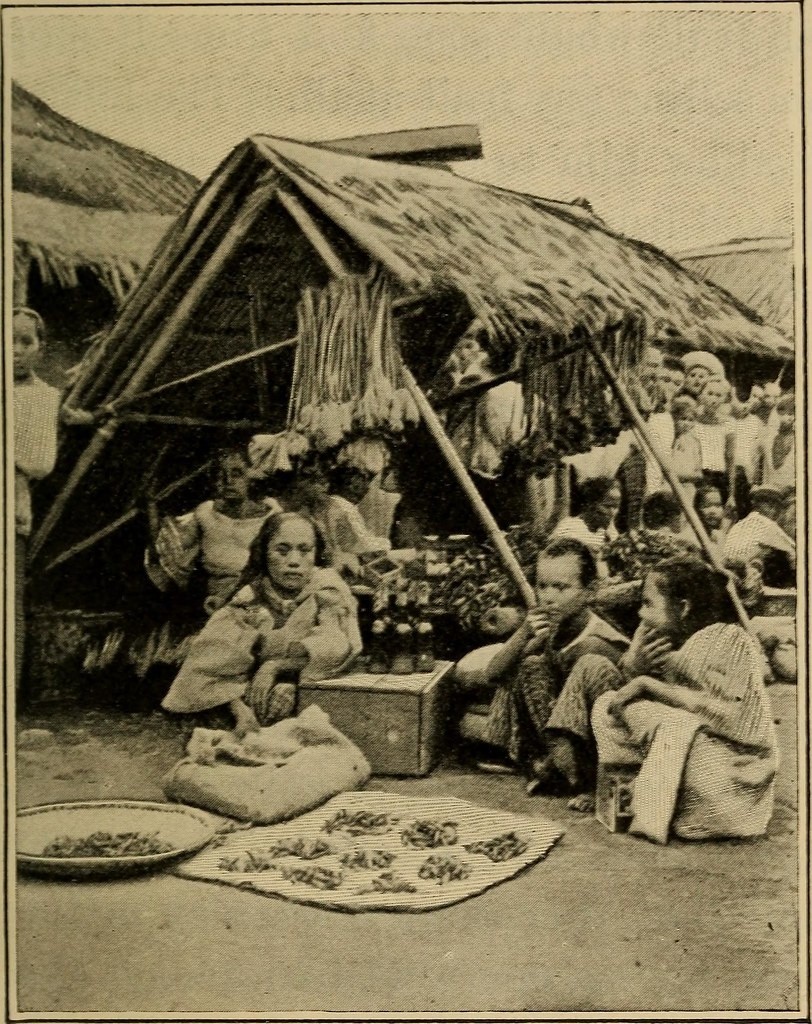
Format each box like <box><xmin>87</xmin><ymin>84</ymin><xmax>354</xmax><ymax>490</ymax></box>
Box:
<box><xmin>477</xmin><ymin>755</ymin><xmax>522</xmax><ymax>772</ymax></box>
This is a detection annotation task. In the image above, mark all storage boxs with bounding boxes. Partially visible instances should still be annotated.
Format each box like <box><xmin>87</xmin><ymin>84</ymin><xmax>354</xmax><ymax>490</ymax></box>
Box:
<box><xmin>298</xmin><ymin>656</ymin><xmax>456</xmax><ymax>780</ymax></box>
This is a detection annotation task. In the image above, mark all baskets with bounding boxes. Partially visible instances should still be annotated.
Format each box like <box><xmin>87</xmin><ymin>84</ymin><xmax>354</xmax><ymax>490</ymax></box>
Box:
<box><xmin>15</xmin><ymin>799</ymin><xmax>216</xmax><ymax>881</ymax></box>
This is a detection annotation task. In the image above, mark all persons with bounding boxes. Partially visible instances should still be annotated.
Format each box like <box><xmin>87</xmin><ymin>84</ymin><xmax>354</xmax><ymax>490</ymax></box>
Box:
<box><xmin>159</xmin><ymin>510</ymin><xmax>361</xmax><ymax>740</ymax></box>
<box><xmin>437</xmin><ymin>314</ymin><xmax>794</xmax><ymax>618</ymax></box>
<box><xmin>133</xmin><ymin>445</ymin><xmax>394</xmax><ymax>618</ymax></box>
<box><xmin>474</xmin><ymin>538</ymin><xmax>631</xmax><ymax>813</ymax></box>
<box><xmin>589</xmin><ymin>554</ymin><xmax>781</xmax><ymax>846</ymax></box>
<box><xmin>13</xmin><ymin>307</ymin><xmax>63</xmax><ymax>692</ymax></box>
